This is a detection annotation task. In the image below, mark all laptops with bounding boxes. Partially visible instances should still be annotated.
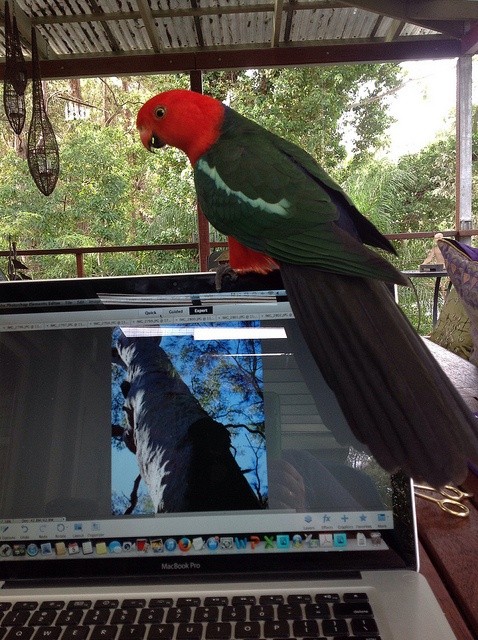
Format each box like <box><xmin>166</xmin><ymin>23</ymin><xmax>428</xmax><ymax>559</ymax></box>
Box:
<box><xmin>0</xmin><ymin>270</ymin><xmax>455</xmax><ymax>636</ymax></box>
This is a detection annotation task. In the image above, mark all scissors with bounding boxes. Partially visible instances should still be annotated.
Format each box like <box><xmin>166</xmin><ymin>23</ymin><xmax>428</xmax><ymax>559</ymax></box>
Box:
<box><xmin>413</xmin><ymin>484</ymin><xmax>473</xmax><ymax>518</ymax></box>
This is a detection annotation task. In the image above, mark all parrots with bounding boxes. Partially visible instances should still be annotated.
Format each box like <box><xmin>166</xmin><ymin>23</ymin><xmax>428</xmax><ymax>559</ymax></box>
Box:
<box><xmin>134</xmin><ymin>87</ymin><xmax>478</xmax><ymax>494</ymax></box>
